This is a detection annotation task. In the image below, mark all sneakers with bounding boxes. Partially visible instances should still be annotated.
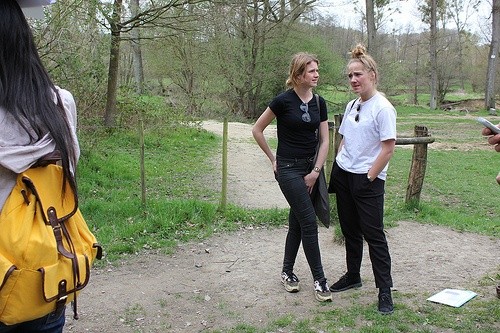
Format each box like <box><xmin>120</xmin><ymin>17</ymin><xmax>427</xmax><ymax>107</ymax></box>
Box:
<box><xmin>314</xmin><ymin>278</ymin><xmax>332</xmax><ymax>302</ymax></box>
<box><xmin>282</xmin><ymin>273</ymin><xmax>300</xmax><ymax>293</ymax></box>
<box><xmin>328</xmin><ymin>274</ymin><xmax>362</xmax><ymax>291</ymax></box>
<box><xmin>378</xmin><ymin>289</ymin><xmax>393</xmax><ymax>314</ymax></box>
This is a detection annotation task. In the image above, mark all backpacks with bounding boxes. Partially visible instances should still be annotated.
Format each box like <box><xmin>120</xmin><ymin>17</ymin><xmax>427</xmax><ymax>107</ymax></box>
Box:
<box><xmin>0</xmin><ymin>142</ymin><xmax>102</xmax><ymax>326</ymax></box>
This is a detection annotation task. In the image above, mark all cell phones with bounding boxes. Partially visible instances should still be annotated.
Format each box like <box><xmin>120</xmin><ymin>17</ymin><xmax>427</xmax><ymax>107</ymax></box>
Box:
<box><xmin>476</xmin><ymin>116</ymin><xmax>500</xmax><ymax>134</ymax></box>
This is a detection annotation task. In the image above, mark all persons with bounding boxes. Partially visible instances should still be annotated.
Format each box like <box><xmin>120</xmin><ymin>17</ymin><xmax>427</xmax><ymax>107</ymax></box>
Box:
<box><xmin>252</xmin><ymin>54</ymin><xmax>333</xmax><ymax>302</ymax></box>
<box><xmin>329</xmin><ymin>45</ymin><xmax>397</xmax><ymax>314</ymax></box>
<box><xmin>0</xmin><ymin>0</ymin><xmax>79</xmax><ymax>333</ymax></box>
<box><xmin>483</xmin><ymin>124</ymin><xmax>500</xmax><ymax>184</ymax></box>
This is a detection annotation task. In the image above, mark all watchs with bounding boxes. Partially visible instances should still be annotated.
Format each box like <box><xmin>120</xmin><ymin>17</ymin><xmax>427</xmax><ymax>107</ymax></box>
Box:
<box><xmin>312</xmin><ymin>167</ymin><xmax>321</xmax><ymax>173</ymax></box>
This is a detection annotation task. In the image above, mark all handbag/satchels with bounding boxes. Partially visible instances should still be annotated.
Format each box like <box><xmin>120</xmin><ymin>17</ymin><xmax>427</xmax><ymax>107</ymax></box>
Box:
<box><xmin>309</xmin><ymin>166</ymin><xmax>330</xmax><ymax>227</ymax></box>
<box><xmin>328</xmin><ymin>167</ymin><xmax>336</xmax><ymax>194</ymax></box>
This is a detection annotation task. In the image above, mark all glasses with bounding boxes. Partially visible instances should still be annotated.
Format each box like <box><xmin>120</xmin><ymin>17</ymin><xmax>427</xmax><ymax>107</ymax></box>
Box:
<box><xmin>355</xmin><ymin>104</ymin><xmax>361</xmax><ymax>122</ymax></box>
